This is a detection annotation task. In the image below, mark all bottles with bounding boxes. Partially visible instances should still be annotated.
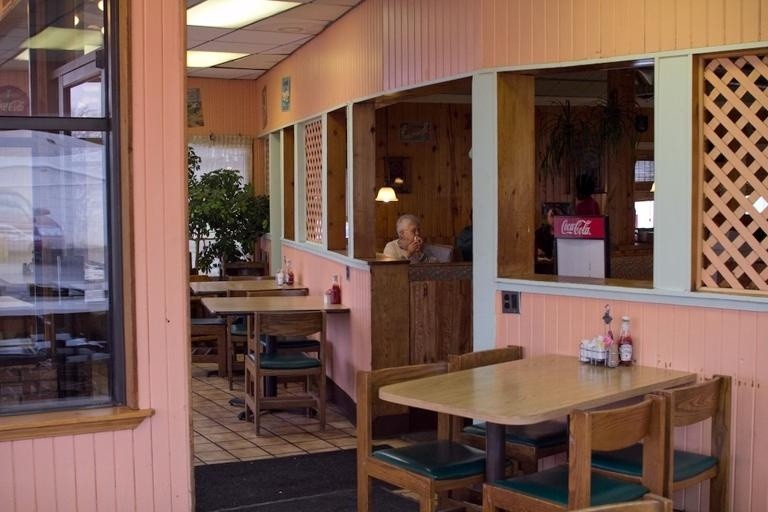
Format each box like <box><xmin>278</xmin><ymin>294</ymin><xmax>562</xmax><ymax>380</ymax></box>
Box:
<box><xmin>323</xmin><ymin>274</ymin><xmax>341</xmax><ymax>306</ymax></box>
<box><xmin>275</xmin><ymin>260</ymin><xmax>295</xmax><ymax>286</ymax></box>
<box><xmin>606</xmin><ymin>316</ymin><xmax>634</xmax><ymax>368</ymax></box>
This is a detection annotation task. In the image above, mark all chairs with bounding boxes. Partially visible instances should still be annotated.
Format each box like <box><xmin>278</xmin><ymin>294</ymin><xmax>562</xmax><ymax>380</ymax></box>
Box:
<box><xmin>446</xmin><ymin>346</ymin><xmax>567</xmax><ymax>474</ymax></box>
<box><xmin>355</xmin><ymin>362</ymin><xmax>518</xmax><ymax>512</ymax></box>
<box><xmin>592</xmin><ymin>371</ymin><xmax>733</xmax><ymax>510</ymax></box>
<box><xmin>190</xmin><ymin>275</ymin><xmax>327</xmax><ymax>436</ymax></box>
<box><xmin>481</xmin><ymin>396</ymin><xmax>673</xmax><ymax>512</ymax></box>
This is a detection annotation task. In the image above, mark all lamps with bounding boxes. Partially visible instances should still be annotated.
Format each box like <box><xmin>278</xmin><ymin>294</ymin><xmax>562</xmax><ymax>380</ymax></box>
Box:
<box><xmin>375</xmin><ymin>107</ymin><xmax>399</xmax><ymax>203</ymax></box>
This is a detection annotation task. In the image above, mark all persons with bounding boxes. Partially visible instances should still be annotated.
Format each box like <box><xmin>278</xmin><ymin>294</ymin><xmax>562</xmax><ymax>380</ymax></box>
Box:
<box><xmin>383</xmin><ymin>214</ymin><xmax>436</xmax><ymax>264</ymax></box>
<box><xmin>448</xmin><ymin>209</ymin><xmax>473</xmax><ymax>263</ymax></box>
<box><xmin>574</xmin><ymin>177</ymin><xmax>600</xmax><ymax>216</ymax></box>
<box><xmin>534</xmin><ymin>206</ymin><xmax>563</xmax><ymax>262</ymax></box>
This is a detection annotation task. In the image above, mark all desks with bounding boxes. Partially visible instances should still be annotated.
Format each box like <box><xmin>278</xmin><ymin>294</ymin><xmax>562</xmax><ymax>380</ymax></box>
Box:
<box><xmin>378</xmin><ymin>353</ymin><xmax>696</xmax><ymax>512</ymax></box>
<box><xmin>200</xmin><ymin>296</ymin><xmax>349</xmax><ymax>421</ymax></box>
